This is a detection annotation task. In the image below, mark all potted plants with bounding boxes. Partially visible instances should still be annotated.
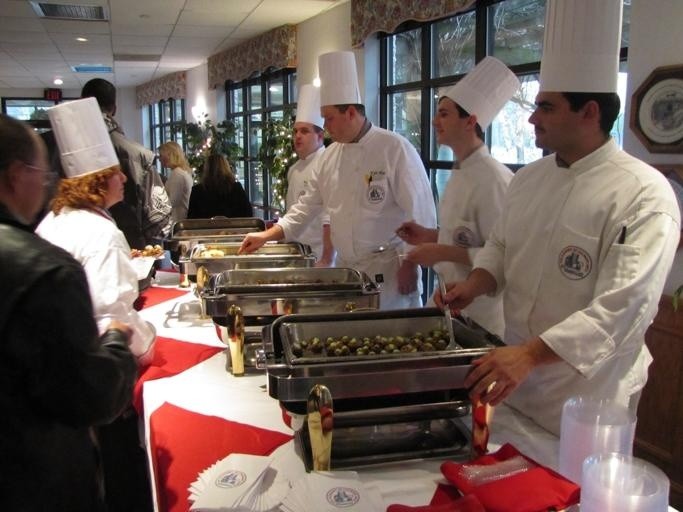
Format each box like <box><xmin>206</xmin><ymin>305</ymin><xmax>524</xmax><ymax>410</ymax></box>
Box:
<box><xmin>167</xmin><ymin>114</ymin><xmax>244</xmax><ymax>186</ymax></box>
<box><xmin>254</xmin><ymin>105</ymin><xmax>328</xmax><ymax>224</ymax></box>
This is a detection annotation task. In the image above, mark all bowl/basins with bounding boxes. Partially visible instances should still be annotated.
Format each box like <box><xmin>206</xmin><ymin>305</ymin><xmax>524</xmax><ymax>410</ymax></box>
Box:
<box><xmin>176</xmin><ymin>228</ymin><xmax>260</xmax><ymax>238</ymax></box>
<box><xmin>220</xmin><ymin>266</ymin><xmax>360</xmax><ymax>287</ymax></box>
<box><xmin>194</xmin><ymin>242</ymin><xmax>305</xmax><ymax>258</ymax></box>
<box><xmin>279</xmin><ymin>314</ymin><xmax>489</xmax><ymax>363</ymax></box>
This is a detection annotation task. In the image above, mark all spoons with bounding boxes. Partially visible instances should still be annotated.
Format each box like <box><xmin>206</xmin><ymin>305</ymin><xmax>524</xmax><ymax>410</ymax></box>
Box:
<box><xmin>435</xmin><ymin>271</ymin><xmax>462</xmax><ymax>353</ymax></box>
<box><xmin>373</xmin><ymin>218</ymin><xmax>417</xmax><ymax>255</ymax></box>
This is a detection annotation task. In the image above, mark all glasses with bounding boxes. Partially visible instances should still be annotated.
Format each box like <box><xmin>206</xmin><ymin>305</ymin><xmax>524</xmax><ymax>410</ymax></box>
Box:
<box><xmin>24</xmin><ymin>163</ymin><xmax>58</xmax><ymax>186</ymax></box>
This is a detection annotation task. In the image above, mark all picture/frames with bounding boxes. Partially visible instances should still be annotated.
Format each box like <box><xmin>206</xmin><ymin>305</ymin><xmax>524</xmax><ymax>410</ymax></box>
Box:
<box><xmin>629</xmin><ymin>63</ymin><xmax>683</xmax><ymax>154</ymax></box>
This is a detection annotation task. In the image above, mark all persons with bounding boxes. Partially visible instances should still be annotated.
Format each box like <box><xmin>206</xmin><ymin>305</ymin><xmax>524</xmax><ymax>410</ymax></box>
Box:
<box><xmin>434</xmin><ymin>0</ymin><xmax>683</xmax><ymax>448</ymax></box>
<box><xmin>34</xmin><ymin>95</ymin><xmax>156</xmax><ymax>369</ymax></box>
<box><xmin>37</xmin><ymin>78</ymin><xmax>172</xmax><ymax>269</ymax></box>
<box><xmin>0</xmin><ymin>114</ymin><xmax>138</xmax><ymax>512</ymax></box>
<box><xmin>235</xmin><ymin>52</ymin><xmax>438</xmax><ymax>310</ymax></box>
<box><xmin>278</xmin><ymin>84</ymin><xmax>336</xmax><ymax>270</ymax></box>
<box><xmin>190</xmin><ymin>153</ymin><xmax>255</xmax><ymax>219</ymax></box>
<box><xmin>396</xmin><ymin>54</ymin><xmax>522</xmax><ymax>341</ymax></box>
<box><xmin>151</xmin><ymin>142</ymin><xmax>194</xmax><ymax>241</ymax></box>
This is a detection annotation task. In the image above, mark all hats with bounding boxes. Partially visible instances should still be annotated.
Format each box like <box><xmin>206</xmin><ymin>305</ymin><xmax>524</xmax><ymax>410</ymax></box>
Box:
<box><xmin>537</xmin><ymin>0</ymin><xmax>623</xmax><ymax>93</ymax></box>
<box><xmin>440</xmin><ymin>56</ymin><xmax>522</xmax><ymax>133</ymax></box>
<box><xmin>296</xmin><ymin>85</ymin><xmax>325</xmax><ymax>130</ymax></box>
<box><xmin>318</xmin><ymin>52</ymin><xmax>362</xmax><ymax>106</ymax></box>
<box><xmin>46</xmin><ymin>97</ymin><xmax>121</xmax><ymax>178</ymax></box>
